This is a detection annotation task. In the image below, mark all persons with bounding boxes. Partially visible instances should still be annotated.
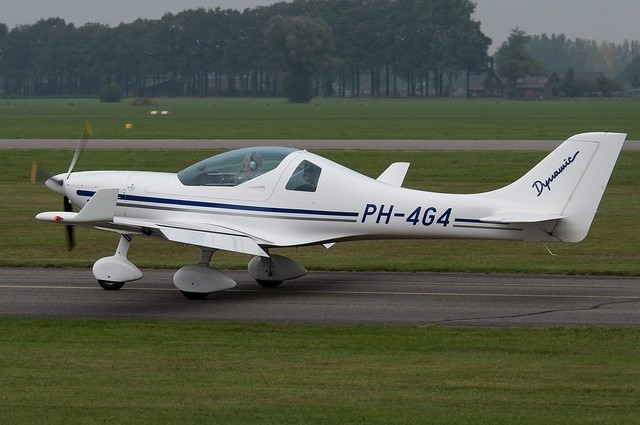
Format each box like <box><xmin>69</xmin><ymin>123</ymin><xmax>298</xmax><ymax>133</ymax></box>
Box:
<box><xmin>239</xmin><ymin>152</ymin><xmax>264</xmax><ymax>185</ymax></box>
<box><xmin>295</xmin><ymin>161</ymin><xmax>320</xmax><ymax>191</ymax></box>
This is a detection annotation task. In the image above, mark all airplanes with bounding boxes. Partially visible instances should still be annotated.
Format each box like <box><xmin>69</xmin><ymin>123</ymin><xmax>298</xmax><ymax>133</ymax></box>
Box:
<box><xmin>31</xmin><ymin>119</ymin><xmax>627</xmax><ymax>301</ymax></box>
